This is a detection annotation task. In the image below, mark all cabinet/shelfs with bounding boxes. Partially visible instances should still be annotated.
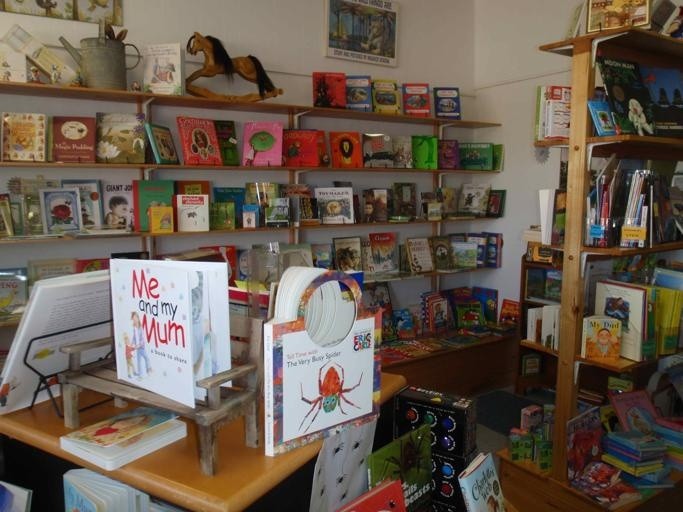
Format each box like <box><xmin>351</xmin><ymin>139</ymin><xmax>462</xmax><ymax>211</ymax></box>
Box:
<box><xmin>498</xmin><ymin>31</ymin><xmax>680</xmax><ymax>510</ymax></box>
<box><xmin>2</xmin><ymin>83</ymin><xmax>499</xmax><ymax>280</ymax></box>
<box><xmin>379</xmin><ymin>308</ymin><xmax>514</xmax><ymax>394</ymax></box>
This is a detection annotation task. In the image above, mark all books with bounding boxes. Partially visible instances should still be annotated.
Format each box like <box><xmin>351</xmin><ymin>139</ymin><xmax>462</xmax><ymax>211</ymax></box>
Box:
<box><xmin>332</xmin><ymin>477</ymin><xmax>406</xmax><ymax>512</ymax></box>
<box><xmin>456</xmin><ymin>451</ymin><xmax>507</xmax><ymax>511</ymax></box>
<box><xmin>0</xmin><ymin>268</ymin><xmax>112</xmax><ymax>412</ymax></box>
<box><xmin>141</xmin><ymin>43</ymin><xmax>183</xmax><ymax>97</ymax></box>
<box><xmin>1</xmin><ymin>23</ymin><xmax>77</xmax><ymax>86</ymax></box>
<box><xmin>58</xmin><ymin>406</ymin><xmax>188</xmax><ymax>473</ymax></box>
<box><xmin>0</xmin><ymin>481</ymin><xmax>35</xmax><ymax>512</ymax></box>
<box><xmin>366</xmin><ymin>422</ymin><xmax>436</xmax><ymax>511</ymax></box>
<box><xmin>61</xmin><ymin>466</ymin><xmax>150</xmax><ymax>511</ymax></box>
<box><xmin>106</xmin><ymin>255</ymin><xmax>233</xmax><ymax>410</ymax></box>
<box><xmin>259</xmin><ymin>264</ymin><xmax>384</xmax><ymax>458</ymax></box>
